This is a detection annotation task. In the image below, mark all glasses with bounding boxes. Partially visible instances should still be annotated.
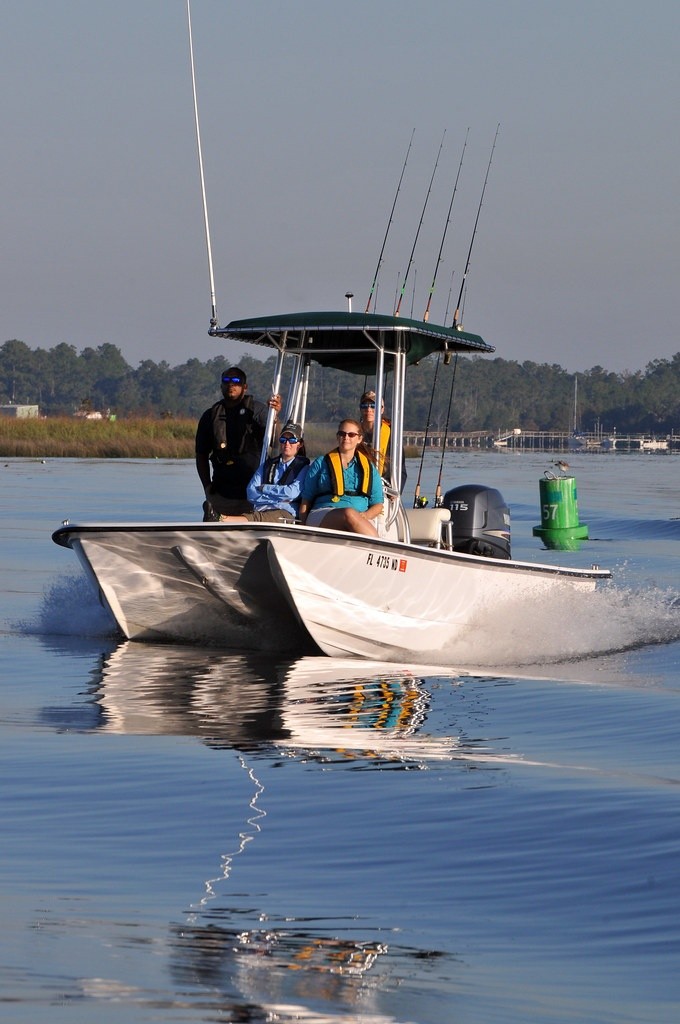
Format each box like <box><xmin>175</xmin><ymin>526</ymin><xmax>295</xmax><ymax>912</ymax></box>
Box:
<box><xmin>222</xmin><ymin>376</ymin><xmax>241</xmax><ymax>383</ymax></box>
<box><xmin>279</xmin><ymin>437</ymin><xmax>300</xmax><ymax>444</ymax></box>
<box><xmin>359</xmin><ymin>402</ymin><xmax>376</xmax><ymax>409</ymax></box>
<box><xmin>336</xmin><ymin>430</ymin><xmax>360</xmax><ymax>438</ymax></box>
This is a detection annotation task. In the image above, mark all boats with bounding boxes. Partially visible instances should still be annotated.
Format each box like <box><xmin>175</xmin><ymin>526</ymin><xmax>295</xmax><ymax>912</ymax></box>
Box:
<box><xmin>638</xmin><ymin>438</ymin><xmax>669</xmax><ymax>455</ymax></box>
<box><xmin>50</xmin><ymin>2</ymin><xmax>680</xmax><ymax>667</ymax></box>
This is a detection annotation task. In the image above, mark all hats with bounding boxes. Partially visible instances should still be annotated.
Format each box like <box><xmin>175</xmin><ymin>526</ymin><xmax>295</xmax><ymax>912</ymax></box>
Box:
<box><xmin>280</xmin><ymin>423</ymin><xmax>304</xmax><ymax>439</ymax></box>
<box><xmin>360</xmin><ymin>390</ymin><xmax>384</xmax><ymax>406</ymax></box>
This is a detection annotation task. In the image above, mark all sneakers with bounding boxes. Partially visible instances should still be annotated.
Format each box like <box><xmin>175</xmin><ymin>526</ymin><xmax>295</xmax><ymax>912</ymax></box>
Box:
<box><xmin>202</xmin><ymin>500</ymin><xmax>221</xmax><ymax>522</ymax></box>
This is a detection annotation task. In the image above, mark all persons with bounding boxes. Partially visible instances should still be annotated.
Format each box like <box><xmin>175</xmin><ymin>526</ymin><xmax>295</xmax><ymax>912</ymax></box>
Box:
<box><xmin>357</xmin><ymin>391</ymin><xmax>408</xmax><ymax>504</ymax></box>
<box><xmin>195</xmin><ymin>367</ymin><xmax>281</xmax><ymax>516</ymax></box>
<box><xmin>203</xmin><ymin>424</ymin><xmax>310</xmax><ymax>524</ymax></box>
<box><xmin>299</xmin><ymin>419</ymin><xmax>384</xmax><ymax>537</ymax></box>
